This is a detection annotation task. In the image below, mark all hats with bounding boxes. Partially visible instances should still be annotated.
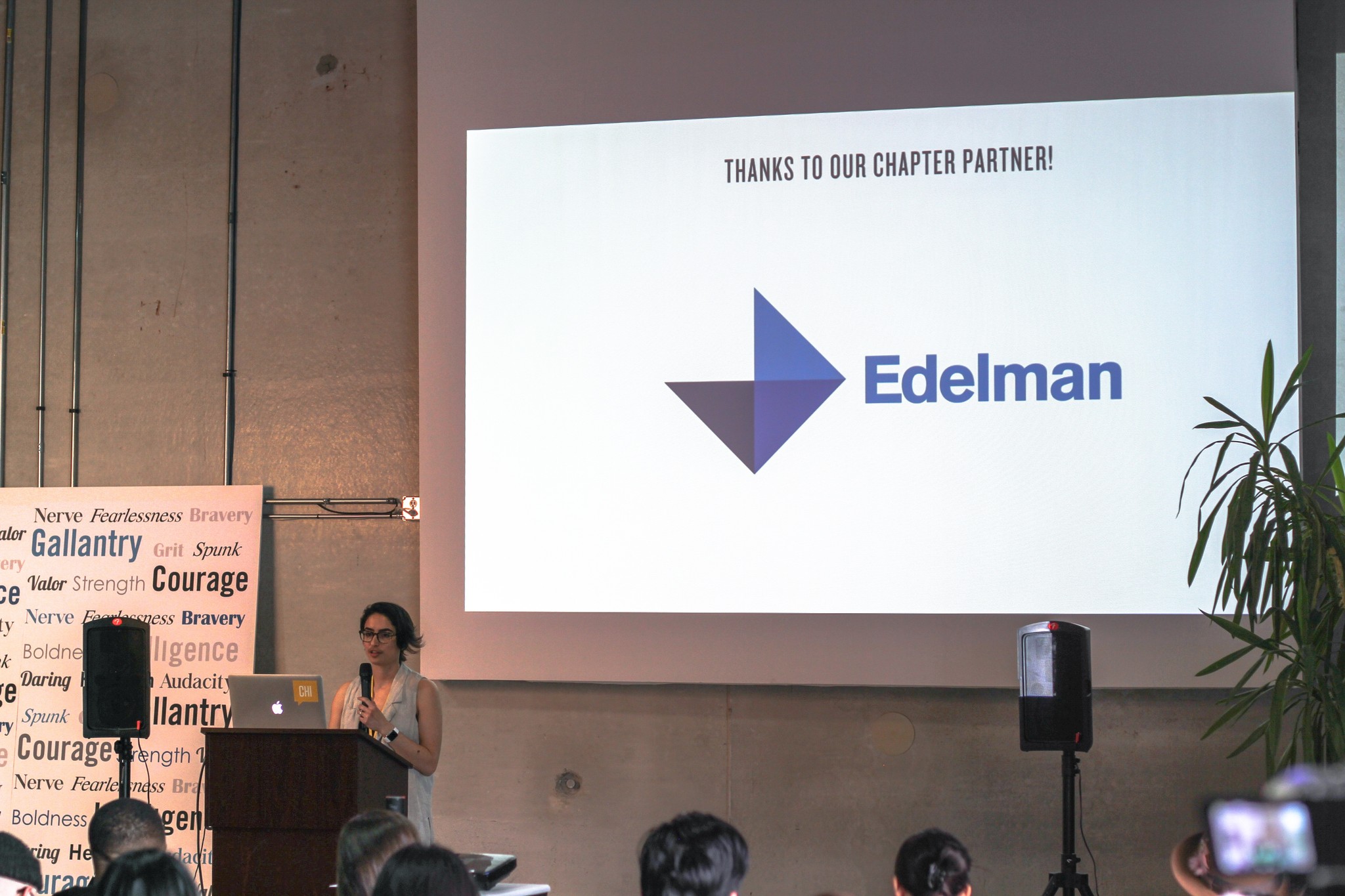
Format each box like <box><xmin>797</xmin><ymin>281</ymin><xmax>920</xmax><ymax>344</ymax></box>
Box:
<box><xmin>0</xmin><ymin>830</ymin><xmax>43</xmax><ymax>891</ymax></box>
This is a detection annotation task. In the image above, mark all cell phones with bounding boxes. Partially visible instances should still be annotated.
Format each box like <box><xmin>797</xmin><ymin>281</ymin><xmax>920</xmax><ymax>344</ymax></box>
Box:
<box><xmin>1200</xmin><ymin>793</ymin><xmax>1324</xmax><ymax>879</ymax></box>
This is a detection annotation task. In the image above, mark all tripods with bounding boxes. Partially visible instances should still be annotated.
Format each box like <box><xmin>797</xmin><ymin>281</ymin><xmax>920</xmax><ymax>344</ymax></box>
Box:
<box><xmin>1039</xmin><ymin>750</ymin><xmax>1093</xmax><ymax>896</ymax></box>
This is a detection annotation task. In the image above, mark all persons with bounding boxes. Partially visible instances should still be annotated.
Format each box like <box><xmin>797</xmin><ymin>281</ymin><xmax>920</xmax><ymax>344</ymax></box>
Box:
<box><xmin>326</xmin><ymin>601</ymin><xmax>443</xmax><ymax>856</ymax></box>
<box><xmin>0</xmin><ymin>797</ymin><xmax>203</xmax><ymax>896</ymax></box>
<box><xmin>334</xmin><ymin>810</ymin><xmax>483</xmax><ymax>896</ymax></box>
<box><xmin>892</xmin><ymin>827</ymin><xmax>974</xmax><ymax>896</ymax></box>
<box><xmin>636</xmin><ymin>810</ymin><xmax>749</xmax><ymax>896</ymax></box>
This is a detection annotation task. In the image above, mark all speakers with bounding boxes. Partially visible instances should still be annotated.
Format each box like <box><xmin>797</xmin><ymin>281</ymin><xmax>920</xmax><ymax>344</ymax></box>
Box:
<box><xmin>81</xmin><ymin>617</ymin><xmax>152</xmax><ymax>738</ymax></box>
<box><xmin>1016</xmin><ymin>619</ymin><xmax>1094</xmax><ymax>752</ymax></box>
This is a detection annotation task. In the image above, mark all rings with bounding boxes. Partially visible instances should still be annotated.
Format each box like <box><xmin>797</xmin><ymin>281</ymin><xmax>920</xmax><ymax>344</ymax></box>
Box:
<box><xmin>359</xmin><ymin>710</ymin><xmax>362</xmax><ymax>715</ymax></box>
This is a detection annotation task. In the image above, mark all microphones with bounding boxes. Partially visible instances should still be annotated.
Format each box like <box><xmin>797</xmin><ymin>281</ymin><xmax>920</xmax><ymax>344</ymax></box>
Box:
<box><xmin>358</xmin><ymin>662</ymin><xmax>373</xmax><ymax>718</ymax></box>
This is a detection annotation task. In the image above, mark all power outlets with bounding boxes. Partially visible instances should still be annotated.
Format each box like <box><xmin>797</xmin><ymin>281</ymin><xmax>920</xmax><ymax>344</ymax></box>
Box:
<box><xmin>401</xmin><ymin>496</ymin><xmax>421</xmax><ymax>522</ymax></box>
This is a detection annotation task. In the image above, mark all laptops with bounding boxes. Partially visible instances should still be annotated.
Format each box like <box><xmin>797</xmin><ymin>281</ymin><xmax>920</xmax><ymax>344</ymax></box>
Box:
<box><xmin>227</xmin><ymin>674</ymin><xmax>328</xmax><ymax>729</ymax></box>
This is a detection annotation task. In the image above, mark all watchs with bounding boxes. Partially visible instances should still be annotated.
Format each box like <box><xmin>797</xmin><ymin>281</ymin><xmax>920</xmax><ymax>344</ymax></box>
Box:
<box><xmin>382</xmin><ymin>727</ymin><xmax>399</xmax><ymax>742</ymax></box>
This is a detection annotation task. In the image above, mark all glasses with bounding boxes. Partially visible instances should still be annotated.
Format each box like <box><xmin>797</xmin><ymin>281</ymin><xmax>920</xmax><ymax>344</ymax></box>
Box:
<box><xmin>359</xmin><ymin>630</ymin><xmax>400</xmax><ymax>644</ymax></box>
